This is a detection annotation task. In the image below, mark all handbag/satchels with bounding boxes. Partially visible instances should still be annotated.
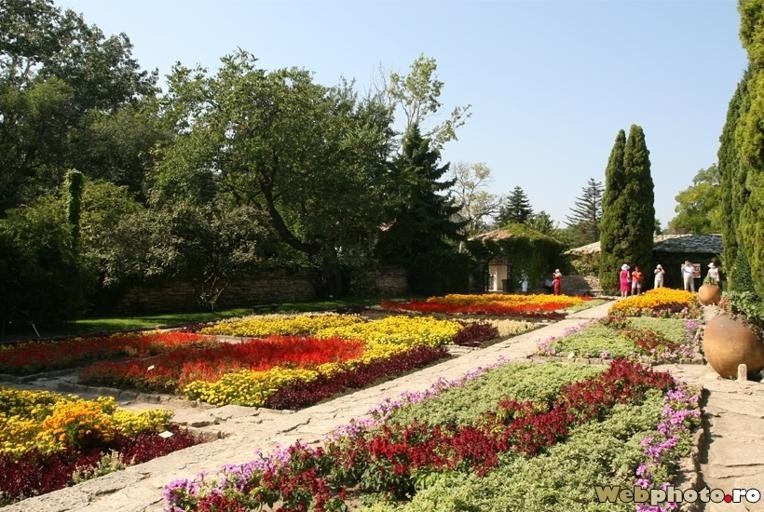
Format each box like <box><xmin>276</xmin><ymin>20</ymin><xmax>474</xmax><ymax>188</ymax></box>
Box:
<box><xmin>627</xmin><ymin>278</ymin><xmax>632</xmax><ymax>283</ymax></box>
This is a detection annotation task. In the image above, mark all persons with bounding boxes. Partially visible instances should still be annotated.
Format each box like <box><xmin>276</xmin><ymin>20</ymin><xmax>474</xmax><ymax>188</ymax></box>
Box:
<box><xmin>653</xmin><ymin>264</ymin><xmax>665</xmax><ymax>290</ymax></box>
<box><xmin>551</xmin><ymin>268</ymin><xmax>563</xmax><ymax>296</ymax></box>
<box><xmin>706</xmin><ymin>262</ymin><xmax>719</xmax><ymax>285</ymax></box>
<box><xmin>631</xmin><ymin>265</ymin><xmax>643</xmax><ymax>296</ymax></box>
<box><xmin>518</xmin><ymin>270</ymin><xmax>528</xmax><ymax>293</ymax></box>
<box><xmin>680</xmin><ymin>260</ymin><xmax>696</xmax><ymax>291</ymax></box>
<box><xmin>619</xmin><ymin>263</ymin><xmax>630</xmax><ymax>298</ymax></box>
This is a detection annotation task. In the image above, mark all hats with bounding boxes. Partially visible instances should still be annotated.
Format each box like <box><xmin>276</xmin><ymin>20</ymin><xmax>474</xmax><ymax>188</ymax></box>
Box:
<box><xmin>656</xmin><ymin>264</ymin><xmax>662</xmax><ymax>269</ymax></box>
<box><xmin>708</xmin><ymin>262</ymin><xmax>716</xmax><ymax>268</ymax></box>
<box><xmin>621</xmin><ymin>263</ymin><xmax>631</xmax><ymax>271</ymax></box>
